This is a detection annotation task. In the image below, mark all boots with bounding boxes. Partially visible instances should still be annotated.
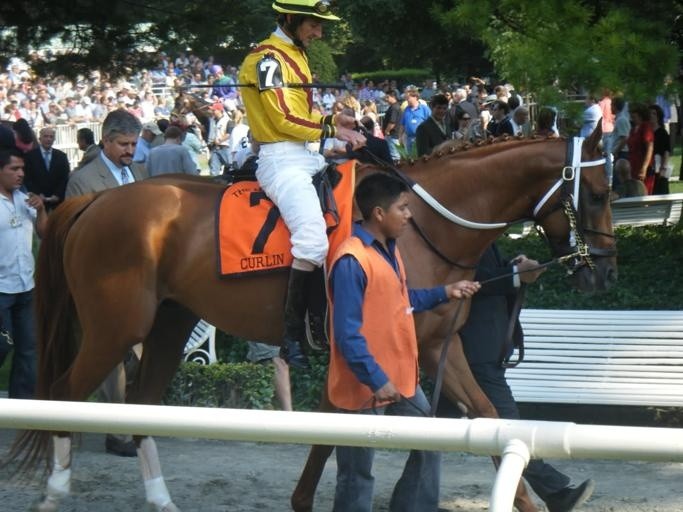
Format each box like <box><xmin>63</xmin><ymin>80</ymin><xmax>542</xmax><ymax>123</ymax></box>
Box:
<box><xmin>276</xmin><ymin>267</ymin><xmax>312</xmax><ymax>371</ymax></box>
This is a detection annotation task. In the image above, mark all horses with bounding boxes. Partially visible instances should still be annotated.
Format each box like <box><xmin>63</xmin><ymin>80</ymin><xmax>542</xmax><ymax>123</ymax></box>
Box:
<box><xmin>0</xmin><ymin>116</ymin><xmax>619</xmax><ymax>512</ymax></box>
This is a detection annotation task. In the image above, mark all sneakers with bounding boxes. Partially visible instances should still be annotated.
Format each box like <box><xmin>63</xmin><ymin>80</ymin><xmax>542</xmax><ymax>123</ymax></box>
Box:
<box><xmin>546</xmin><ymin>477</ymin><xmax>596</xmax><ymax>511</ymax></box>
<box><xmin>103</xmin><ymin>434</ymin><xmax>139</xmax><ymax>457</ymax></box>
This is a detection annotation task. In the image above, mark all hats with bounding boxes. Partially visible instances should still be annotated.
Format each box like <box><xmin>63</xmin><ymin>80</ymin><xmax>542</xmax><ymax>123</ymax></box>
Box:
<box><xmin>208</xmin><ymin>65</ymin><xmax>222</xmax><ymax>75</ymax></box>
<box><xmin>270</xmin><ymin>1</ymin><xmax>342</xmax><ymax>22</ymax></box>
<box><xmin>208</xmin><ymin>102</ymin><xmax>224</xmax><ymax>111</ymax></box>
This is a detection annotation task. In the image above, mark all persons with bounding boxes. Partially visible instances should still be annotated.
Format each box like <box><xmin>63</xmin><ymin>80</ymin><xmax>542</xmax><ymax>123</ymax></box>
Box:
<box><xmin>1</xmin><ymin>49</ymin><xmax>256</xmax><ymax>175</ymax></box>
<box><xmin>62</xmin><ymin>104</ymin><xmax>149</xmax><ymax>459</ymax></box>
<box><xmin>383</xmin><ymin>240</ymin><xmax>595</xmax><ymax>512</ymax></box>
<box><xmin>311</xmin><ymin>73</ymin><xmax>556</xmax><ymax>164</ymax></box>
<box><xmin>0</xmin><ymin>146</ymin><xmax>48</xmax><ymax>400</ymax></box>
<box><xmin>322</xmin><ymin>172</ymin><xmax>482</xmax><ymax>512</ymax></box>
<box><xmin>578</xmin><ymin>75</ymin><xmax>681</xmax><ymax>200</ymax></box>
<box><xmin>242</xmin><ymin>334</ymin><xmax>293</xmax><ymax>410</ymax></box>
<box><xmin>235</xmin><ymin>0</ymin><xmax>369</xmax><ymax>372</ymax></box>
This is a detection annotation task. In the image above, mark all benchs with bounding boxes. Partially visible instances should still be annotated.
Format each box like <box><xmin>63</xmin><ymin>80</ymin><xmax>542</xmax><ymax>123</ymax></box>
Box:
<box><xmin>507</xmin><ymin>193</ymin><xmax>683</xmax><ymax>240</ymax></box>
<box><xmin>504</xmin><ymin>310</ymin><xmax>682</xmax><ymax>408</ymax></box>
<box><xmin>133</xmin><ymin>324</ymin><xmax>218</xmax><ymax>372</ymax></box>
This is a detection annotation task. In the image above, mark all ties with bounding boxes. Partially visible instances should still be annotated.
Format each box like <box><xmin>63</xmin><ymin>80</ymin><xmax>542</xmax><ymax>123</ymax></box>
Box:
<box><xmin>120</xmin><ymin>167</ymin><xmax>131</xmax><ymax>185</ymax></box>
<box><xmin>43</xmin><ymin>150</ymin><xmax>51</xmax><ymax>169</ymax></box>
